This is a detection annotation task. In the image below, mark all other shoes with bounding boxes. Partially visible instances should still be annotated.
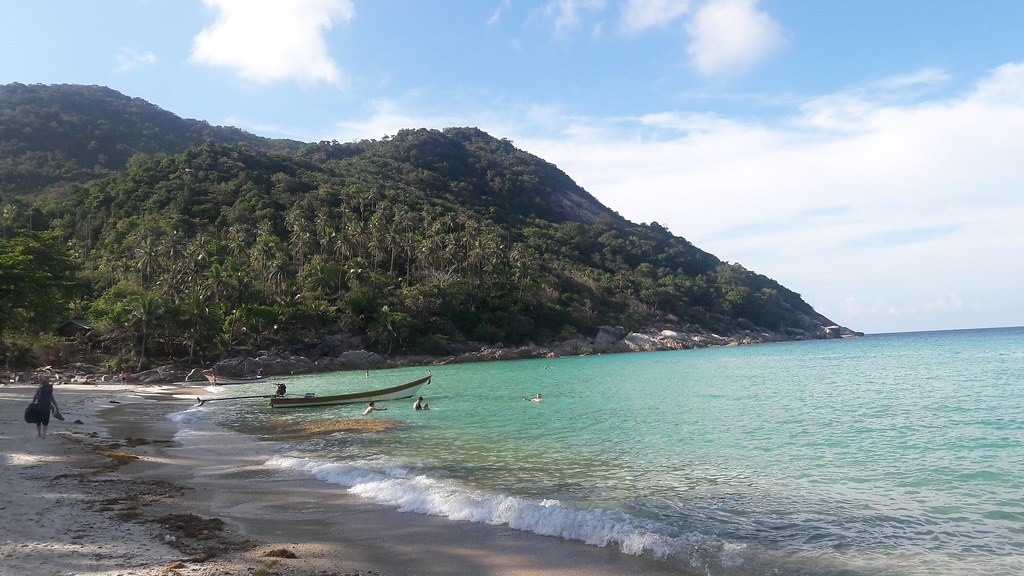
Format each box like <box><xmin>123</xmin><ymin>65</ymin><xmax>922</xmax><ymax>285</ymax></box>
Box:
<box><xmin>52</xmin><ymin>411</ymin><xmax>66</xmax><ymax>422</ymax></box>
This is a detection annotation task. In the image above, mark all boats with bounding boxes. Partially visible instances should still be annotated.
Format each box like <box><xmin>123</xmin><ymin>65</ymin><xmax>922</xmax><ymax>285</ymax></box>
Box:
<box><xmin>203</xmin><ymin>370</ymin><xmax>268</xmax><ymax>385</ymax></box>
<box><xmin>270</xmin><ymin>369</ymin><xmax>433</xmax><ymax>407</ymax></box>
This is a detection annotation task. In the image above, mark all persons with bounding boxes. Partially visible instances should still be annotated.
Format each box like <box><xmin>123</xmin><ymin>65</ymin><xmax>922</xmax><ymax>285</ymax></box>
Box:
<box><xmin>413</xmin><ymin>396</ymin><xmax>423</xmax><ymax>409</ymax></box>
<box><xmin>33</xmin><ymin>375</ymin><xmax>60</xmax><ymax>439</ymax></box>
<box><xmin>364</xmin><ymin>401</ymin><xmax>387</xmax><ymax>414</ymax></box>
<box><xmin>536</xmin><ymin>393</ymin><xmax>541</xmax><ymax>399</ymax></box>
<box><xmin>212</xmin><ymin>374</ymin><xmax>216</xmax><ymax>387</ymax></box>
<box><xmin>121</xmin><ymin>371</ymin><xmax>127</xmax><ymax>385</ymax></box>
<box><xmin>422</xmin><ymin>404</ymin><xmax>429</xmax><ymax>409</ymax></box>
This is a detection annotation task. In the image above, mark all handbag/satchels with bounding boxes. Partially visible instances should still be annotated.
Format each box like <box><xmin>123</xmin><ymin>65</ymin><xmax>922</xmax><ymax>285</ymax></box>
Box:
<box><xmin>24</xmin><ymin>404</ymin><xmax>40</xmax><ymax>424</ymax></box>
